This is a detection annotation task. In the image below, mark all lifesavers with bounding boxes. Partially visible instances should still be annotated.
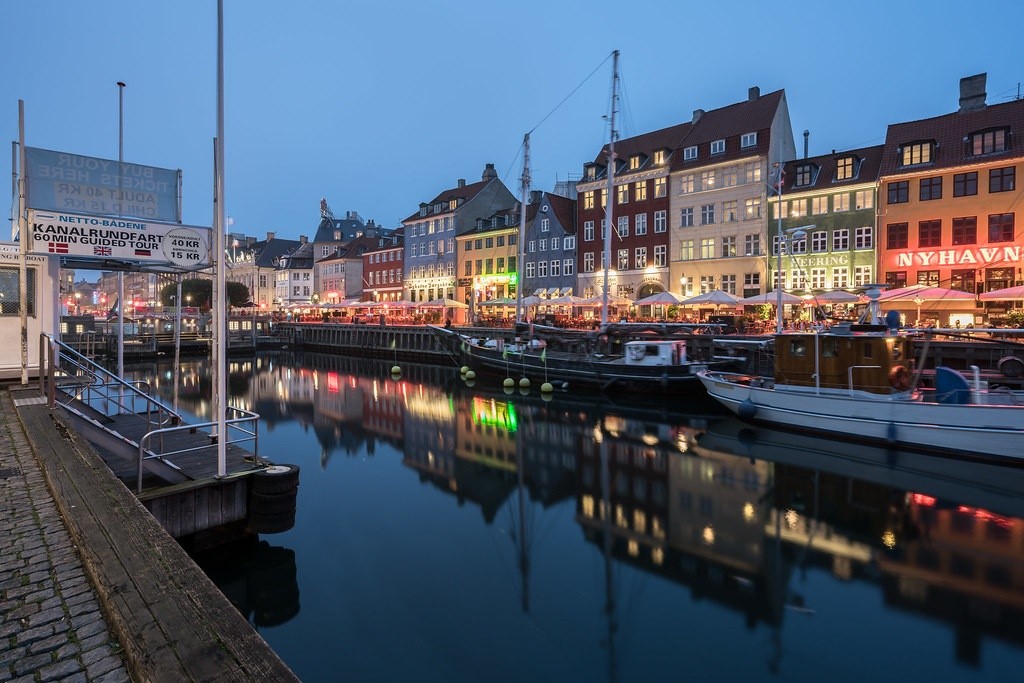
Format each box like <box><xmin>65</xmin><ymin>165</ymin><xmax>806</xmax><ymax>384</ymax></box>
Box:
<box><xmin>888</xmin><ymin>365</ymin><xmax>914</xmax><ymax>391</ymax></box>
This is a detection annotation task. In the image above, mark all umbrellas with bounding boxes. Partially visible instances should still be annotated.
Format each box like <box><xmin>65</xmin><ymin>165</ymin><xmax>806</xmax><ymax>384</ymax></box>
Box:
<box><xmin>282</xmin><ymin>297</ymin><xmax>470</xmax><ymax>326</ymax></box>
<box><xmin>866</xmin><ymin>285</ymin><xmax>977</xmax><ymax>301</ymax></box>
<box><xmin>979</xmin><ymin>285</ymin><xmax>1024</xmax><ymax>302</ymax></box>
<box><xmin>476</xmin><ymin>286</ymin><xmax>860</xmax><ymax>316</ymax></box>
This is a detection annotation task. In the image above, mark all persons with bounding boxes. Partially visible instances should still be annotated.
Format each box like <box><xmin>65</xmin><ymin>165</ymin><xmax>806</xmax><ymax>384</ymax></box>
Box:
<box><xmin>488</xmin><ymin>310</ymin><xmax>858</xmax><ymax>335</ymax></box>
<box><xmin>898</xmin><ymin>318</ymin><xmax>1024</xmax><ymax>343</ymax></box>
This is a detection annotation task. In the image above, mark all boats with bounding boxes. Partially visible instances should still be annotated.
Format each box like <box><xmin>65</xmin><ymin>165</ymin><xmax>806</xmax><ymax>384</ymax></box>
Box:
<box><xmin>436</xmin><ymin>376</ymin><xmax>709</xmax><ymax>415</ymax></box>
<box><xmin>696</xmin><ymin>331</ymin><xmax>1023</xmax><ymax>461</ymax></box>
<box><xmin>695</xmin><ymin>418</ymin><xmax>1022</xmax><ymax>518</ymax></box>
<box><xmin>428</xmin><ymin>323</ymin><xmax>743</xmax><ymax>388</ymax></box>
